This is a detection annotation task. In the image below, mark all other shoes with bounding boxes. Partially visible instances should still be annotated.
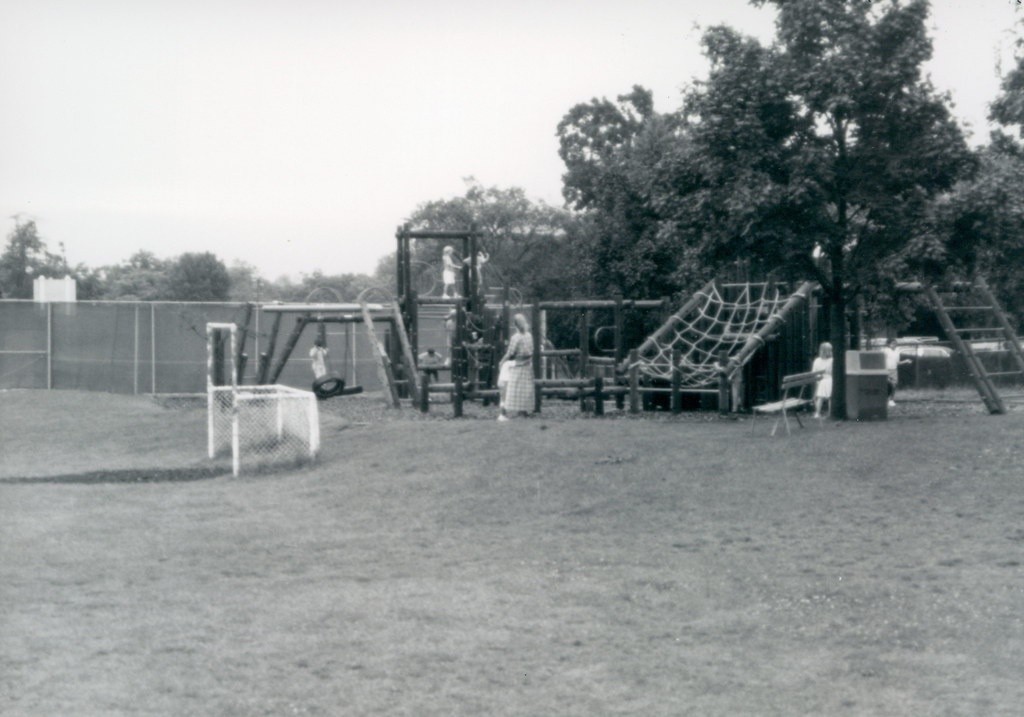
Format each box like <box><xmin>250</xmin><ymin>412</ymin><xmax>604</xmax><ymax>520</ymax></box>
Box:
<box><xmin>889</xmin><ymin>400</ymin><xmax>896</xmax><ymax>407</ymax></box>
<box><xmin>814</xmin><ymin>413</ymin><xmax>820</xmax><ymax>418</ymax></box>
<box><xmin>498</xmin><ymin>414</ymin><xmax>509</xmax><ymax>422</ymax></box>
<box><xmin>453</xmin><ymin>293</ymin><xmax>461</xmax><ymax>298</ymax></box>
<box><xmin>442</xmin><ymin>294</ymin><xmax>449</xmax><ymax>299</ymax></box>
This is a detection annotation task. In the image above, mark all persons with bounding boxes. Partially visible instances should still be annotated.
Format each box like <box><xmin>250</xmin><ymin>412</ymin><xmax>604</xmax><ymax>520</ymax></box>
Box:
<box><xmin>464</xmin><ymin>246</ymin><xmax>489</xmax><ymax>295</ymax></box>
<box><xmin>496</xmin><ymin>312</ymin><xmax>535</xmax><ymax>423</ymax></box>
<box><xmin>441</xmin><ymin>245</ymin><xmax>463</xmax><ymax>299</ymax></box>
<box><xmin>812</xmin><ymin>343</ymin><xmax>834</xmax><ymax>419</ymax></box>
<box><xmin>309</xmin><ymin>338</ymin><xmax>331</xmax><ymax>381</ymax></box>
<box><xmin>878</xmin><ymin>337</ymin><xmax>912</xmax><ymax>407</ymax></box>
<box><xmin>419</xmin><ymin>347</ymin><xmax>443</xmax><ymax>383</ymax></box>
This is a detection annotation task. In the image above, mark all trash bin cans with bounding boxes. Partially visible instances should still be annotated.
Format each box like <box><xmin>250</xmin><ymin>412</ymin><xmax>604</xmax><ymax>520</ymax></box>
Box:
<box><xmin>846</xmin><ymin>349</ymin><xmax>887</xmax><ymax>421</ymax></box>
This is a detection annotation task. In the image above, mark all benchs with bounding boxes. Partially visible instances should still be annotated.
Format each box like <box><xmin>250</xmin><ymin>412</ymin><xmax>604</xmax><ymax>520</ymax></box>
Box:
<box><xmin>750</xmin><ymin>369</ymin><xmax>827</xmax><ymax>438</ymax></box>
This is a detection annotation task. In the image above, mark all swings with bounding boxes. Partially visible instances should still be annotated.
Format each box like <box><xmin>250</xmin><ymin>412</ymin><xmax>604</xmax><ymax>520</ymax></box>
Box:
<box><xmin>310</xmin><ymin>311</ymin><xmax>344</xmax><ymax>398</ymax></box>
